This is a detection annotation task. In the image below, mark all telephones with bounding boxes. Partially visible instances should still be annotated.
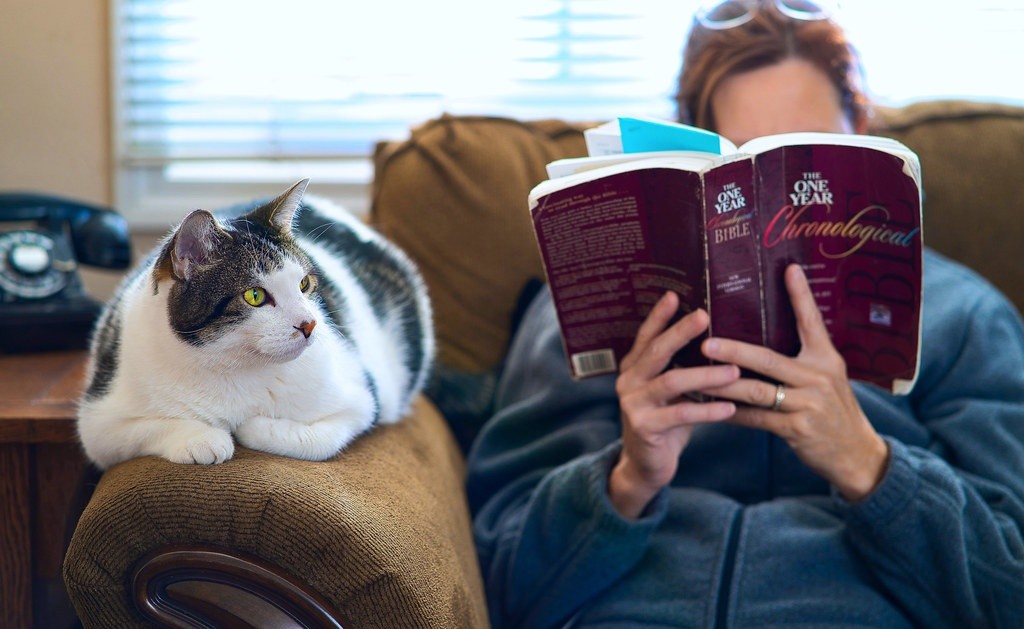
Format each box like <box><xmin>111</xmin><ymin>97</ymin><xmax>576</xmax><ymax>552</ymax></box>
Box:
<box><xmin>0</xmin><ymin>190</ymin><xmax>133</xmax><ymax>356</ymax></box>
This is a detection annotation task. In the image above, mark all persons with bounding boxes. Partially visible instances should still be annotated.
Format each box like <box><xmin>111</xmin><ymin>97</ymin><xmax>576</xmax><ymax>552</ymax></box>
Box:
<box><xmin>456</xmin><ymin>0</ymin><xmax>1024</xmax><ymax>629</ymax></box>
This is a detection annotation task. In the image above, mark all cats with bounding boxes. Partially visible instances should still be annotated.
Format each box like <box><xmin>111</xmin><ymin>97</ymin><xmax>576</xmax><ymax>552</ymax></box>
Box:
<box><xmin>72</xmin><ymin>177</ymin><xmax>437</xmax><ymax>466</ymax></box>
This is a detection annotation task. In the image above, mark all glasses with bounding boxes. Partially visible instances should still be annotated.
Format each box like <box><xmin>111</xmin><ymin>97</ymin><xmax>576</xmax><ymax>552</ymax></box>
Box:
<box><xmin>689</xmin><ymin>0</ymin><xmax>842</xmax><ymax>32</ymax></box>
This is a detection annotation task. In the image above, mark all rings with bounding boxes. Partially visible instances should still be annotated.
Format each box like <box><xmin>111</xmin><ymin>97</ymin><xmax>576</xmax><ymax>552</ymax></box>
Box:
<box><xmin>771</xmin><ymin>384</ymin><xmax>785</xmax><ymax>411</ymax></box>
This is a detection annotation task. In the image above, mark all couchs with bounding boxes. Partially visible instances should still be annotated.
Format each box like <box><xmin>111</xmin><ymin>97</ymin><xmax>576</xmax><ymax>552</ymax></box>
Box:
<box><xmin>63</xmin><ymin>102</ymin><xmax>1024</xmax><ymax>629</ymax></box>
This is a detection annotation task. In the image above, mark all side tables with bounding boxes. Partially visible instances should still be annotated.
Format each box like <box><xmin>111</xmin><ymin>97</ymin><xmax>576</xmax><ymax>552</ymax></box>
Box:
<box><xmin>0</xmin><ymin>351</ymin><xmax>103</xmax><ymax>629</ymax></box>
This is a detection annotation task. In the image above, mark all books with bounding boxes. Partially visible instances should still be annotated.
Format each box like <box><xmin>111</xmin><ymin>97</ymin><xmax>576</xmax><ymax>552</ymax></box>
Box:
<box><xmin>527</xmin><ymin>117</ymin><xmax>923</xmax><ymax>397</ymax></box>
